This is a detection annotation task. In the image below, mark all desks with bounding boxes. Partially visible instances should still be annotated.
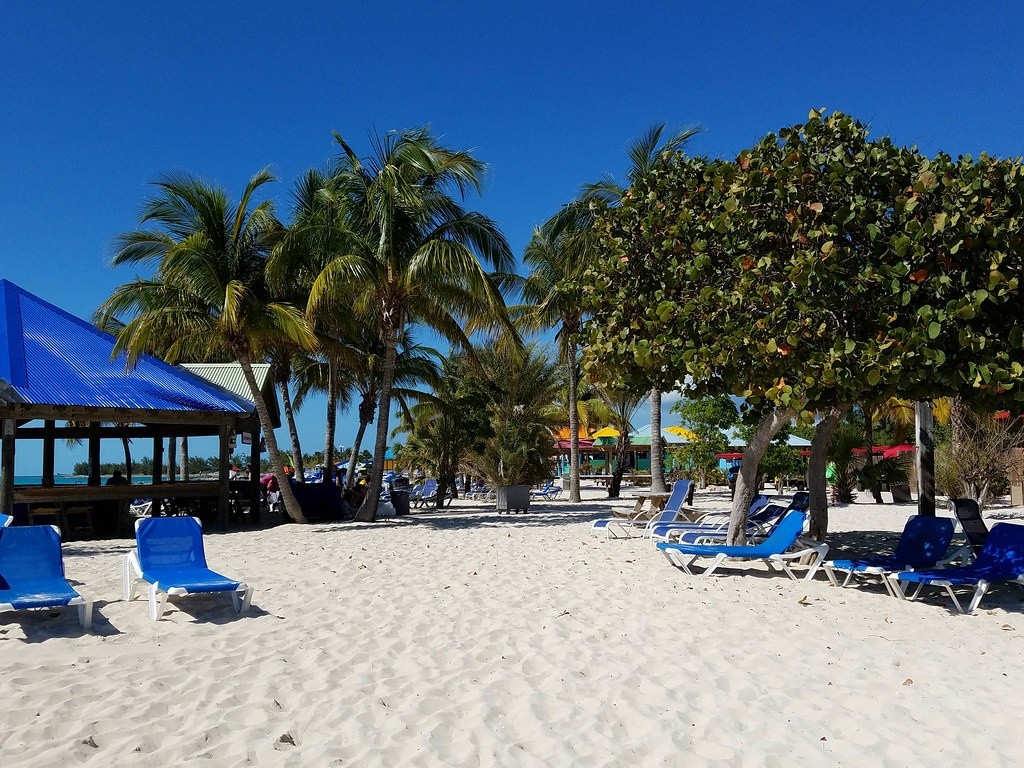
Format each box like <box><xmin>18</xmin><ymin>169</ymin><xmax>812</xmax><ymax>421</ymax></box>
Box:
<box><xmin>631</xmin><ymin>492</ymin><xmax>671</xmax><ymax>510</ymax></box>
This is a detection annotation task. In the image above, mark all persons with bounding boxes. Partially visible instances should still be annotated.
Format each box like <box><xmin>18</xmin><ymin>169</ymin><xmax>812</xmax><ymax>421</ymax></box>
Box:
<box><xmin>465</xmin><ymin>477</ymin><xmax>471</xmax><ymax>494</ymax></box>
<box><xmin>105</xmin><ymin>469</ymin><xmax>127</xmax><ymax>485</ymax></box>
<box><xmin>260</xmin><ymin>465</ymin><xmax>295</xmax><ymax>512</ymax></box>
<box><xmin>472</xmin><ymin>477</ymin><xmax>483</xmax><ymax>487</ymax></box>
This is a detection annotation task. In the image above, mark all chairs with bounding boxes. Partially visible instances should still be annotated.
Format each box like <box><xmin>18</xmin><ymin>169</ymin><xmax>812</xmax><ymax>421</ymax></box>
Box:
<box><xmin>457</xmin><ymin>480</ymin><xmax>561</xmax><ymax>501</ymax></box>
<box><xmin>0</xmin><ymin>525</ymin><xmax>94</xmax><ymax>630</ymax></box>
<box><xmin>27</xmin><ymin>503</ymin><xmax>62</xmax><ymax>528</ymax></box>
<box><xmin>592</xmin><ymin>478</ymin><xmax>1024</xmax><ymax>618</ymax></box>
<box><xmin>60</xmin><ymin>503</ymin><xmax>94</xmax><ymax>534</ymax></box>
<box><xmin>122</xmin><ymin>516</ymin><xmax>254</xmax><ymax>622</ymax></box>
<box><xmin>408</xmin><ymin>479</ymin><xmax>453</xmax><ymax>511</ymax></box>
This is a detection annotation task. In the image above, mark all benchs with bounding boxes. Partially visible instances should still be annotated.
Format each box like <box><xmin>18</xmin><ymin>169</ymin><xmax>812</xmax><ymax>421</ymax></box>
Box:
<box><xmin>676</xmin><ymin>506</ymin><xmax>710</xmax><ymax>521</ymax></box>
<box><xmin>611</xmin><ymin>506</ymin><xmax>639</xmax><ymax>520</ymax></box>
